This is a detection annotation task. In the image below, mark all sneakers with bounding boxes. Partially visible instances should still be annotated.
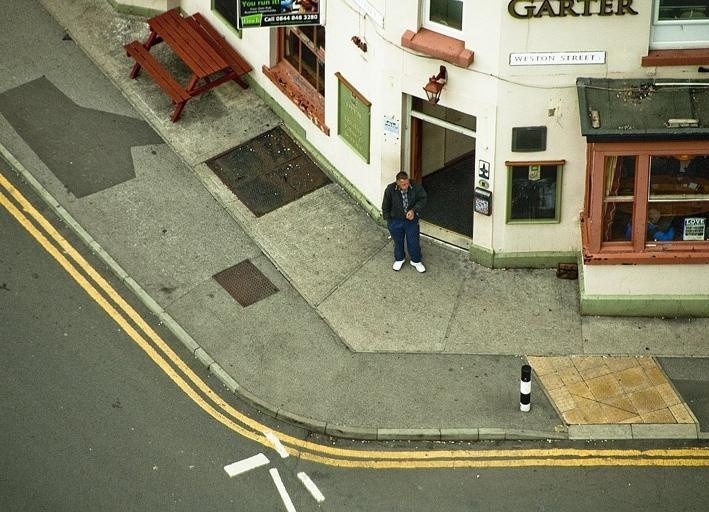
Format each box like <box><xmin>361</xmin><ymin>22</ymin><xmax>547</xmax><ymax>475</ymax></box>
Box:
<box><xmin>393</xmin><ymin>259</ymin><xmax>405</xmax><ymax>271</ymax></box>
<box><xmin>410</xmin><ymin>260</ymin><xmax>426</xmax><ymax>273</ymax></box>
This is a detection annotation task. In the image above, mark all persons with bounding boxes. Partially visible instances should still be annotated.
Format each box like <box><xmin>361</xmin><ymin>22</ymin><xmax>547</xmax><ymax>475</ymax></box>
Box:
<box><xmin>626</xmin><ymin>209</ymin><xmax>676</xmax><ymax>240</ymax></box>
<box><xmin>380</xmin><ymin>170</ymin><xmax>431</xmax><ymax>275</ymax></box>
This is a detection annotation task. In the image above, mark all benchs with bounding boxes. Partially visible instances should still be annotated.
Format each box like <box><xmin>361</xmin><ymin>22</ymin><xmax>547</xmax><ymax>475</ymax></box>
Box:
<box><xmin>185</xmin><ymin>12</ymin><xmax>252</xmax><ymax>77</ymax></box>
<box><xmin>123</xmin><ymin>40</ymin><xmax>192</xmax><ymax>104</ymax></box>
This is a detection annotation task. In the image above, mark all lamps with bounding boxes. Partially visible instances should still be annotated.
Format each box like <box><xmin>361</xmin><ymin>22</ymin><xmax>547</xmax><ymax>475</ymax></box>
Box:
<box><xmin>422</xmin><ymin>65</ymin><xmax>447</xmax><ymax>107</ymax></box>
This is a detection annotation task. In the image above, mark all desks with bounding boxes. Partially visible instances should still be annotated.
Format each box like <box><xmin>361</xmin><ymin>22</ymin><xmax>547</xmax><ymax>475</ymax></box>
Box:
<box><xmin>616</xmin><ymin>174</ymin><xmax>709</xmax><ymax>230</ymax></box>
<box><xmin>130</xmin><ymin>9</ymin><xmax>249</xmax><ymax>123</ymax></box>
